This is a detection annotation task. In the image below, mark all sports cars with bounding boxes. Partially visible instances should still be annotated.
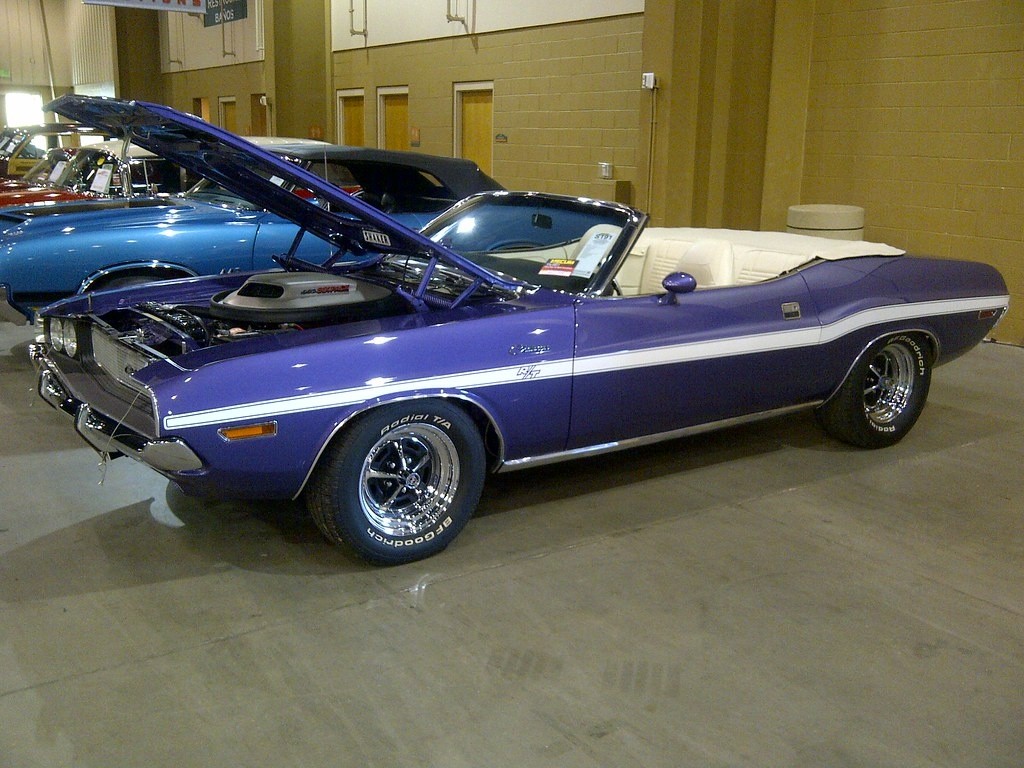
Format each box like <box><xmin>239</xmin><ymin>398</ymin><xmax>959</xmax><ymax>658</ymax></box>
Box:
<box><xmin>29</xmin><ymin>92</ymin><xmax>1013</xmax><ymax>570</ymax></box>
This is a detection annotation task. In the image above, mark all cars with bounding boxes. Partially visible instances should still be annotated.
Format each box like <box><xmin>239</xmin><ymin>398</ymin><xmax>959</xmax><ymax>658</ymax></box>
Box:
<box><xmin>0</xmin><ymin>143</ymin><xmax>628</xmax><ymax>324</ymax></box>
<box><xmin>0</xmin><ymin>126</ymin><xmax>362</xmax><ymax>208</ymax></box>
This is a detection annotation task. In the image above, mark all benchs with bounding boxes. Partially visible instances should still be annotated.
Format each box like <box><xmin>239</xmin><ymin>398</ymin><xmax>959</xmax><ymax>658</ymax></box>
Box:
<box><xmin>360</xmin><ymin>186</ymin><xmax>435</xmax><ymax>214</ymax></box>
<box><xmin>578</xmin><ymin>223</ymin><xmax>812</xmax><ymax>297</ymax></box>
